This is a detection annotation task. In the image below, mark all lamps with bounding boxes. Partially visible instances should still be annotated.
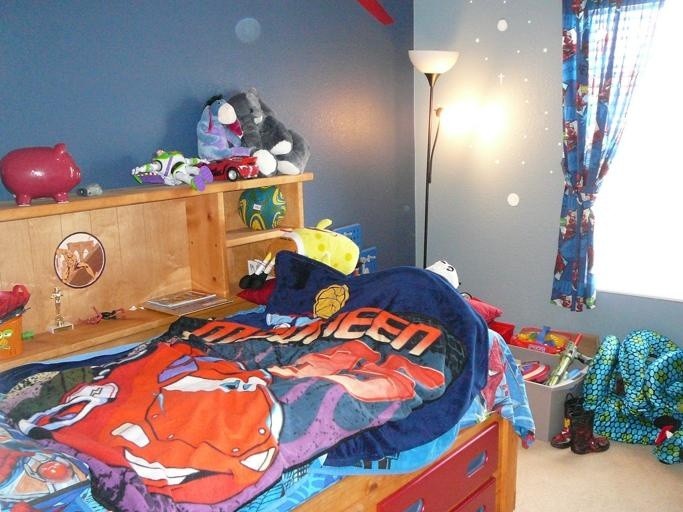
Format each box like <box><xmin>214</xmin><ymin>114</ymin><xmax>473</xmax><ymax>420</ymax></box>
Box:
<box><xmin>406</xmin><ymin>48</ymin><xmax>462</xmax><ymax>272</ymax></box>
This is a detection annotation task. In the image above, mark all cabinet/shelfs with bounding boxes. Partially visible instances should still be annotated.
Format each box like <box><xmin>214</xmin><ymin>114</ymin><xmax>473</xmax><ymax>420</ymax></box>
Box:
<box><xmin>0</xmin><ymin>171</ymin><xmax>317</xmax><ymax>372</ymax></box>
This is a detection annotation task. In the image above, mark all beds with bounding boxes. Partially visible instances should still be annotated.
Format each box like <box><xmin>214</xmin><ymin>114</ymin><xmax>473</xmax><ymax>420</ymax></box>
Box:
<box><xmin>0</xmin><ymin>340</ymin><xmax>519</xmax><ymax>512</ymax></box>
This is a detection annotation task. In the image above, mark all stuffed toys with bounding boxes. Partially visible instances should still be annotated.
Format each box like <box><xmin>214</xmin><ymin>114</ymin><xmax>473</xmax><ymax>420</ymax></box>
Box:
<box><xmin>129</xmin><ymin>86</ymin><xmax>311</xmax><ymax>191</ymax></box>
<box><xmin>239</xmin><ymin>218</ymin><xmax>362</xmax><ymax>290</ymax></box>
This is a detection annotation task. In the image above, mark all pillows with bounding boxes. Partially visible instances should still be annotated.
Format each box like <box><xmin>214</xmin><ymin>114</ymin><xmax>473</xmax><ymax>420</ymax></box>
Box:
<box><xmin>236</xmin><ymin>278</ymin><xmax>276</xmax><ymax>305</ymax></box>
<box><xmin>460</xmin><ymin>297</ymin><xmax>504</xmax><ymax>328</ymax></box>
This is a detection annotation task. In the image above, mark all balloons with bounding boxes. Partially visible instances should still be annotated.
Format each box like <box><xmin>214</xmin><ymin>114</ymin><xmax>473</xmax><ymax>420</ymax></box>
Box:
<box><xmin>581</xmin><ymin>330</ymin><xmax>683</xmax><ymax>464</ymax></box>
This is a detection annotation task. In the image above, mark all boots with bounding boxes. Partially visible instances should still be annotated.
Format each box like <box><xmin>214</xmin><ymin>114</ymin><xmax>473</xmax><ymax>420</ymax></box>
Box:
<box><xmin>570</xmin><ymin>410</ymin><xmax>610</xmax><ymax>454</ymax></box>
<box><xmin>552</xmin><ymin>398</ymin><xmax>583</xmax><ymax>448</ymax></box>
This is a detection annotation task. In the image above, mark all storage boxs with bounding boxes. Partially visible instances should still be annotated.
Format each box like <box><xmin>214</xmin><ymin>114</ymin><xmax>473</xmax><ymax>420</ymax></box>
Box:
<box><xmin>500</xmin><ymin>345</ymin><xmax>589</xmax><ymax>443</ymax></box>
